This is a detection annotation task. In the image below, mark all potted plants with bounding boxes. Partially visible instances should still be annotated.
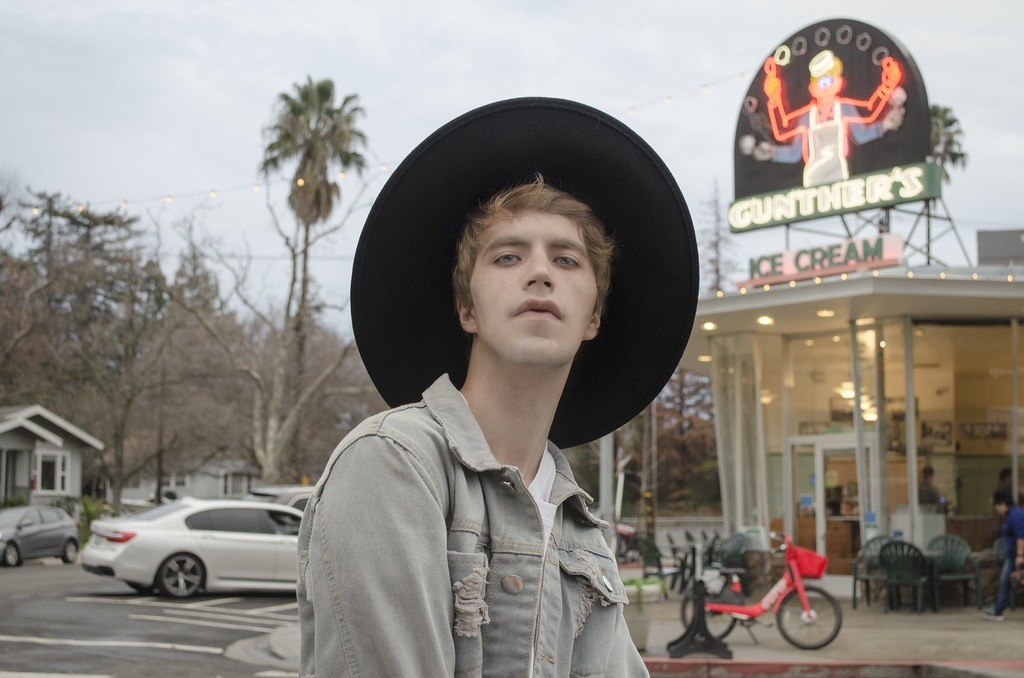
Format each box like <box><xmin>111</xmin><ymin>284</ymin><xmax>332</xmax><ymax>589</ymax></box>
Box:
<box><xmin>622</xmin><ymin>578</ymin><xmax>669</xmax><ymax>651</ymax></box>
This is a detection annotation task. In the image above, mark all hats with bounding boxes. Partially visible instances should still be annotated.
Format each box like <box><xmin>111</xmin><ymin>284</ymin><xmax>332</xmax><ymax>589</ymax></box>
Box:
<box><xmin>349</xmin><ymin>97</ymin><xmax>699</xmax><ymax>448</ymax></box>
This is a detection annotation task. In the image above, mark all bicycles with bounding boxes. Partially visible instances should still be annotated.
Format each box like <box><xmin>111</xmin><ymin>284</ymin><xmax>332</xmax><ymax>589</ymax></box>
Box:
<box><xmin>680</xmin><ymin>531</ymin><xmax>843</xmax><ymax>649</ymax></box>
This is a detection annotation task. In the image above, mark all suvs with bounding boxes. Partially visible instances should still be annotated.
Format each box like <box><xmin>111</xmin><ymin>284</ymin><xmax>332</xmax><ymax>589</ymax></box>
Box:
<box><xmin>242</xmin><ymin>485</ymin><xmax>316</xmax><ymax>512</ymax></box>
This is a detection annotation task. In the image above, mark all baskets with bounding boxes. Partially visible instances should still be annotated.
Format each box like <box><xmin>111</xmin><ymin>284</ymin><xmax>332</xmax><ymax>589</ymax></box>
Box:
<box><xmin>794</xmin><ymin>546</ymin><xmax>828</xmax><ymax>577</ymax></box>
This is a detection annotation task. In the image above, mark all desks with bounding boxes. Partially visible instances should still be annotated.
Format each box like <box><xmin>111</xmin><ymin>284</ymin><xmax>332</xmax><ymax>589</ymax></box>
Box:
<box><xmin>856</xmin><ymin>549</ymin><xmax>945</xmax><ymax>611</ymax></box>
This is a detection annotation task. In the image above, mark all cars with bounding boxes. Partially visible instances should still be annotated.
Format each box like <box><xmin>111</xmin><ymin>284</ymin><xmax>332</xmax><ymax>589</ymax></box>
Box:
<box><xmin>81</xmin><ymin>495</ymin><xmax>305</xmax><ymax>601</ymax></box>
<box><xmin>0</xmin><ymin>505</ymin><xmax>80</xmax><ymax>567</ymax></box>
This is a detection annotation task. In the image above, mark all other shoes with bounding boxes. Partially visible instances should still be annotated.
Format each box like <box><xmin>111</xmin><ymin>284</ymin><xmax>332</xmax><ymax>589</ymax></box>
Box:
<box><xmin>981</xmin><ymin>609</ymin><xmax>1004</xmax><ymax>621</ymax></box>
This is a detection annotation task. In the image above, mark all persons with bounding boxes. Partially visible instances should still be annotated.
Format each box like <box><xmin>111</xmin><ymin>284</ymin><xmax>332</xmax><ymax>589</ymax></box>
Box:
<box><xmin>811</xmin><ymin>486</ymin><xmax>851</xmax><ymax>515</ymax></box>
<box><xmin>993</xmin><ymin>468</ymin><xmax>1013</xmax><ymax>494</ymax></box>
<box><xmin>982</xmin><ymin>493</ymin><xmax>1024</xmax><ymax>621</ymax></box>
<box><xmin>920</xmin><ymin>466</ymin><xmax>939</xmax><ymax>504</ymax></box>
<box><xmin>298</xmin><ymin>97</ymin><xmax>700</xmax><ymax>678</ymax></box>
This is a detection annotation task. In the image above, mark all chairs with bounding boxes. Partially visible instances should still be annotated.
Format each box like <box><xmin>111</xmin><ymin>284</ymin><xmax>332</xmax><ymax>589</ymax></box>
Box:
<box><xmin>853</xmin><ymin>534</ymin><xmax>981</xmax><ymax>612</ymax></box>
<box><xmin>632</xmin><ymin>533</ymin><xmax>752</xmax><ymax>598</ymax></box>
<box><xmin>993</xmin><ymin>538</ymin><xmax>1016</xmax><ymax>610</ymax></box>
